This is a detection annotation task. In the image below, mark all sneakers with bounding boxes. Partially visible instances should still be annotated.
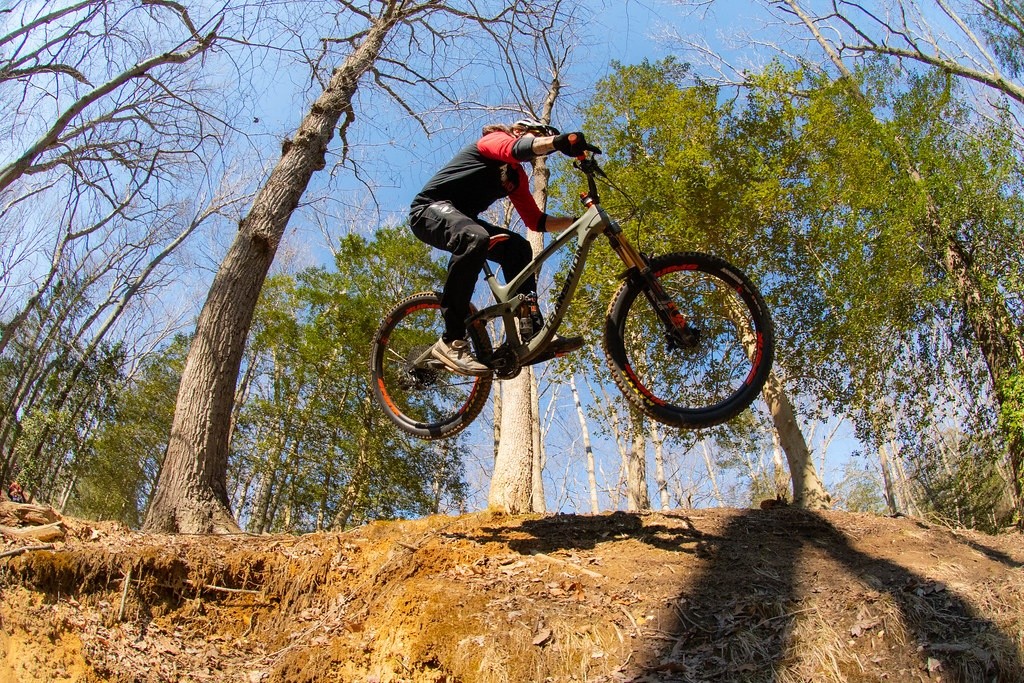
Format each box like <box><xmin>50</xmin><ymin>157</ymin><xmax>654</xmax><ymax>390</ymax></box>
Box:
<box><xmin>544</xmin><ymin>333</ymin><xmax>586</xmax><ymax>353</ymax></box>
<box><xmin>431</xmin><ymin>335</ymin><xmax>490</xmax><ymax>377</ymax></box>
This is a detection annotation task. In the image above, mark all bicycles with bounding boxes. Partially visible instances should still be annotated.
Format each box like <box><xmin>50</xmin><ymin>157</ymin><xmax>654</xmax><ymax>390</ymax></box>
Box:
<box><xmin>370</xmin><ymin>130</ymin><xmax>778</xmax><ymax>441</ymax></box>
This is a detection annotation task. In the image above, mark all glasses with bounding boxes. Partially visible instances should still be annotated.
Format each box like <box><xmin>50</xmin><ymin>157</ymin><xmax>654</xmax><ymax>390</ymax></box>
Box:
<box><xmin>529</xmin><ymin>128</ymin><xmax>549</xmax><ymax>137</ymax></box>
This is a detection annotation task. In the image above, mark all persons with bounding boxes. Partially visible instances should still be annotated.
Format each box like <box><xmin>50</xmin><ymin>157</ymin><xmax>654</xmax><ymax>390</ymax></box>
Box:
<box><xmin>409</xmin><ymin>116</ymin><xmax>587</xmax><ymax>376</ymax></box>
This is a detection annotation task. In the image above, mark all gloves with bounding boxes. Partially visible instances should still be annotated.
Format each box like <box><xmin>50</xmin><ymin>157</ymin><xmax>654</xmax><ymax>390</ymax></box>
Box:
<box><xmin>553</xmin><ymin>132</ymin><xmax>602</xmax><ymax>157</ymax></box>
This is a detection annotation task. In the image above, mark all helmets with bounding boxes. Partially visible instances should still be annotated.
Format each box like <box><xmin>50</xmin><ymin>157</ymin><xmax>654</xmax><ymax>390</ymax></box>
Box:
<box><xmin>516</xmin><ymin>118</ymin><xmax>561</xmax><ymax>155</ymax></box>
